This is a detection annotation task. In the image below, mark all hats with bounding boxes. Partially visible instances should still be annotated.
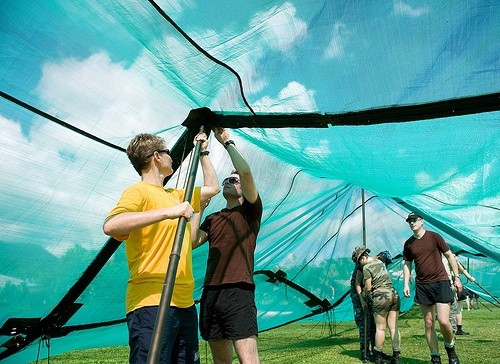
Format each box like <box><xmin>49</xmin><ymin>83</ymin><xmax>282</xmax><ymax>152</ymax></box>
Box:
<box><xmin>379</xmin><ymin>250</ymin><xmax>393</xmax><ymax>268</ymax></box>
<box><xmin>352</xmin><ymin>246</ymin><xmax>371</xmax><ymax>263</ymax></box>
<box><xmin>406</xmin><ymin>212</ymin><xmax>423</xmax><ymax>222</ymax></box>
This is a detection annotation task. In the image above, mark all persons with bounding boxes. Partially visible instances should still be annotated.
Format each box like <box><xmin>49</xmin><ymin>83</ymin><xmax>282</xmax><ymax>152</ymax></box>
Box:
<box><xmin>440</xmin><ymin>250</ymin><xmax>480</xmax><ymax>335</ymax></box>
<box><xmin>350</xmin><ymin>246</ymin><xmax>402</xmax><ymax>364</ymax></box>
<box><xmin>102</xmin><ymin>132</ymin><xmax>222</xmax><ymax>364</ymax></box>
<box><xmin>190</xmin><ymin>128</ymin><xmax>263</xmax><ymax>364</ymax></box>
<box><xmin>402</xmin><ymin>212</ymin><xmax>462</xmax><ymax>364</ymax></box>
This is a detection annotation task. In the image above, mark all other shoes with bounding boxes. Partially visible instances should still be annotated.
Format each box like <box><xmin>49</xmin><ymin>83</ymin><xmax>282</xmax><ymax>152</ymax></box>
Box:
<box><xmin>446</xmin><ymin>346</ymin><xmax>460</xmax><ymax>364</ymax></box>
<box><xmin>391</xmin><ymin>350</ymin><xmax>401</xmax><ymax>364</ymax></box>
<box><xmin>372</xmin><ymin>348</ymin><xmax>382</xmax><ymax>364</ymax></box>
<box><xmin>456</xmin><ymin>329</ymin><xmax>470</xmax><ymax>335</ymax></box>
<box><xmin>430</xmin><ymin>355</ymin><xmax>441</xmax><ymax>364</ymax></box>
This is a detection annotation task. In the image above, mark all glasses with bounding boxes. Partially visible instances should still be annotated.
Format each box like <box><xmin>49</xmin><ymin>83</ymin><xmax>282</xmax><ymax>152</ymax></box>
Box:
<box><xmin>409</xmin><ymin>219</ymin><xmax>417</xmax><ymax>222</ymax></box>
<box><xmin>221</xmin><ymin>176</ymin><xmax>240</xmax><ymax>185</ymax></box>
<box><xmin>146</xmin><ymin>149</ymin><xmax>169</xmax><ymax>161</ymax></box>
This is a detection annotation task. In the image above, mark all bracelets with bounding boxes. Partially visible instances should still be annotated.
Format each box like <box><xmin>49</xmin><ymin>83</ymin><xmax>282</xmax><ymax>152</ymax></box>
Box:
<box><xmin>224</xmin><ymin>140</ymin><xmax>235</xmax><ymax>150</ymax></box>
<box><xmin>199</xmin><ymin>150</ymin><xmax>210</xmax><ymax>156</ymax></box>
